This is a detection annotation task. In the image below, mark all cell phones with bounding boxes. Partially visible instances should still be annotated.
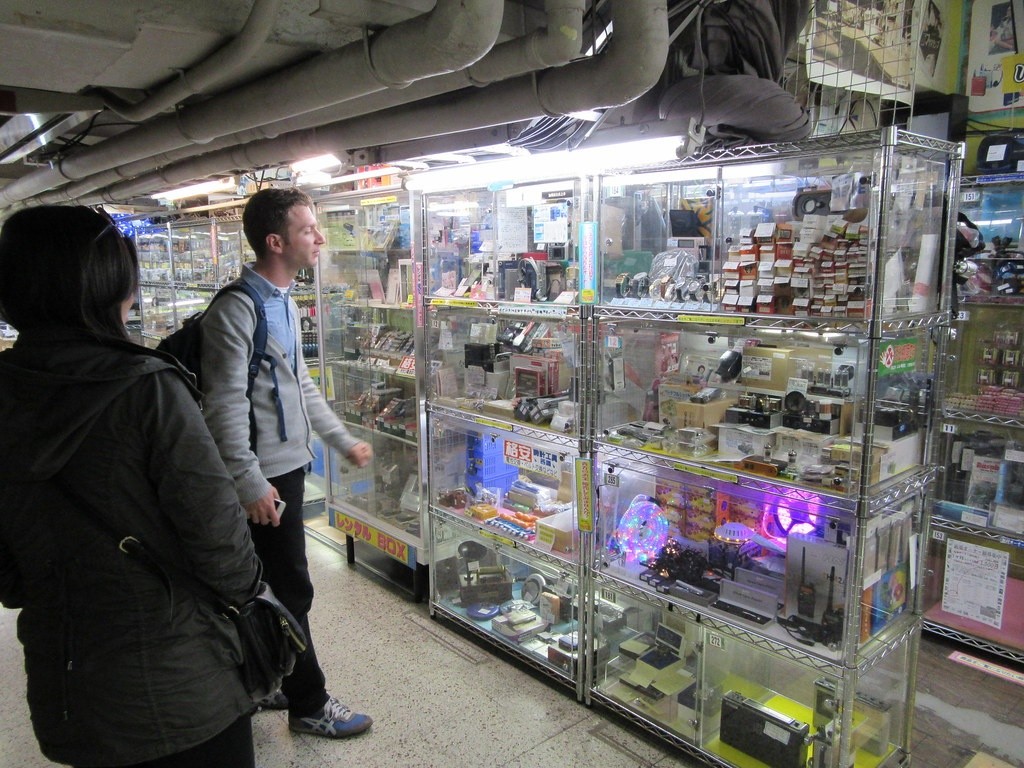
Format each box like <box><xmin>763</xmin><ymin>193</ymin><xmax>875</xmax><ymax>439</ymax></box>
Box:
<box><xmin>267</xmin><ymin>498</ymin><xmax>285</xmax><ymax>521</ymax></box>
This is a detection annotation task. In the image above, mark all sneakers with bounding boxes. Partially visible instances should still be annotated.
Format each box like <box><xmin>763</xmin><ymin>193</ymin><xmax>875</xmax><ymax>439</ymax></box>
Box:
<box><xmin>288</xmin><ymin>697</ymin><xmax>373</xmax><ymax>736</ymax></box>
<box><xmin>254</xmin><ymin>690</ymin><xmax>289</xmax><ymax>710</ymax></box>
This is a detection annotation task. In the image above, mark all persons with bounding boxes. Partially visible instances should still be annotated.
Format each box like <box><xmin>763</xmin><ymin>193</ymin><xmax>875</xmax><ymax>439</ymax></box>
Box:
<box><xmin>200</xmin><ymin>188</ymin><xmax>372</xmax><ymax>736</ymax></box>
<box><xmin>1</xmin><ymin>203</ymin><xmax>261</xmax><ymax>768</ymax></box>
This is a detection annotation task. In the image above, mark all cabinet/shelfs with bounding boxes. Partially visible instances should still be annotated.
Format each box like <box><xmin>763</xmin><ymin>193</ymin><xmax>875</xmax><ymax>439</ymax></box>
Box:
<box><xmin>917</xmin><ymin>171</ymin><xmax>1024</xmax><ymax>662</ymax></box>
<box><xmin>410</xmin><ymin>172</ymin><xmax>659</xmax><ymax>704</ymax></box>
<box><xmin>133</xmin><ymin>219</ymin><xmax>253</xmax><ymax>350</ymax></box>
<box><xmin>311</xmin><ymin>195</ymin><xmax>499</xmax><ymax>599</ymax></box>
<box><xmin>584</xmin><ymin>128</ymin><xmax>969</xmax><ymax>768</ymax></box>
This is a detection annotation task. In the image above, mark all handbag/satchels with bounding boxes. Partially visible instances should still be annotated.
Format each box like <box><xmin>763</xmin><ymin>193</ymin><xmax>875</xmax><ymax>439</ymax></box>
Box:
<box><xmin>223</xmin><ymin>581</ymin><xmax>306</xmax><ymax>704</ymax></box>
<box><xmin>155</xmin><ymin>277</ymin><xmax>287</xmax><ymax>456</ymax></box>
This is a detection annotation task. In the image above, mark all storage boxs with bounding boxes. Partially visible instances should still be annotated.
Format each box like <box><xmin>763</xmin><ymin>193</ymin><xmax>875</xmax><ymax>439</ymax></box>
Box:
<box><xmin>139</xmin><ymin>160</ymin><xmax>1024</xmax><ymax>731</ymax></box>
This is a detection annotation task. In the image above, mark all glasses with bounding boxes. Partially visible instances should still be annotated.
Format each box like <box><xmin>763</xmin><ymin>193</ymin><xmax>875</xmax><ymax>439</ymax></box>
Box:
<box><xmin>92</xmin><ymin>207</ymin><xmax>125</xmax><ymax>243</ymax></box>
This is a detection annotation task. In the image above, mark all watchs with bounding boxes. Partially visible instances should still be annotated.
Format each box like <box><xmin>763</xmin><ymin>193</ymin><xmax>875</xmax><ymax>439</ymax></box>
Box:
<box><xmin>615</xmin><ymin>272</ymin><xmax>706</xmax><ymax>302</ymax></box>
